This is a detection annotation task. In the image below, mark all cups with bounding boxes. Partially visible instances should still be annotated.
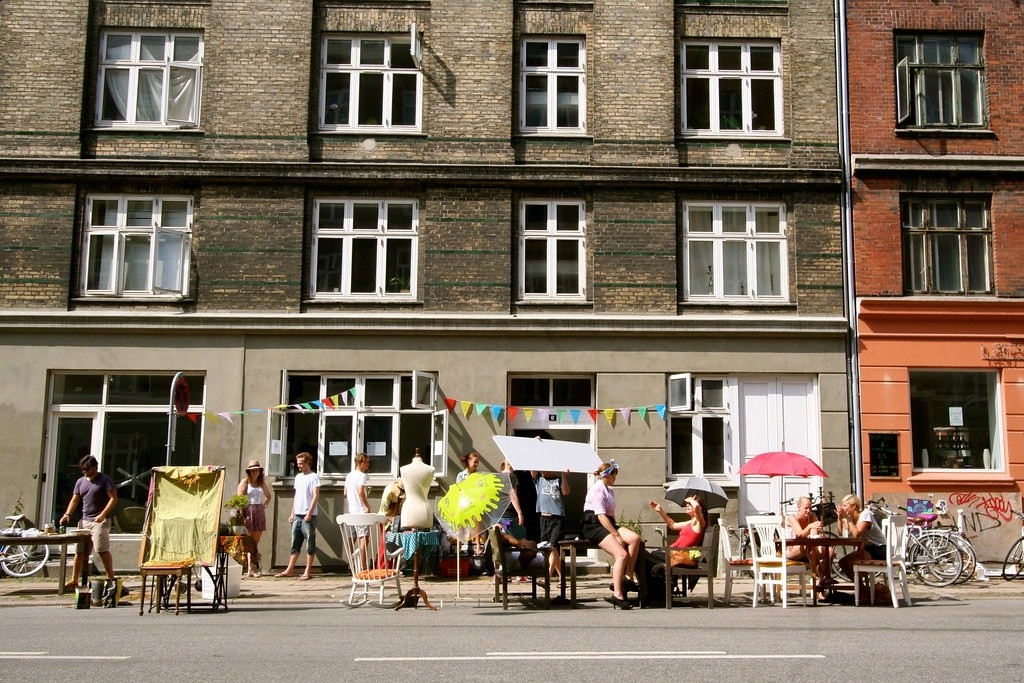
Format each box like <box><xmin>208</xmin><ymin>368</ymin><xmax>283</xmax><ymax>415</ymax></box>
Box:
<box><xmin>810</xmin><ymin>528</ymin><xmax>818</xmax><ymax>535</ymax></box>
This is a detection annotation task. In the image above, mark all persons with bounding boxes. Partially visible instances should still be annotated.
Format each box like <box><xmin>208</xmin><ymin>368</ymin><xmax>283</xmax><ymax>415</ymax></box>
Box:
<box><xmin>343</xmin><ymin>453</ymin><xmax>371</xmax><ymax>571</ymax></box>
<box><xmin>378</xmin><ymin>476</ymin><xmax>406</xmax><ymax>577</ymax></box>
<box><xmin>650</xmin><ymin>495</ymin><xmax>706</xmax><ymax>601</ymax></box>
<box><xmin>237</xmin><ymin>460</ymin><xmax>271</xmax><ymax>576</ymax></box>
<box><xmin>400</xmin><ymin>457</ymin><xmax>435</xmax><ymax>530</ymax></box>
<box><xmin>274</xmin><ymin>452</ymin><xmax>321</xmax><ymax>580</ymax></box>
<box><xmin>490</xmin><ymin>457</ymin><xmax>529</xmax><ymax>585</ymax></box>
<box><xmin>455</xmin><ymin>450</ymin><xmax>484</xmax><ymax>555</ymax></box>
<box><xmin>60</xmin><ymin>455</ymin><xmax>118</xmax><ymax>588</ymax></box>
<box><xmin>581</xmin><ymin>458</ymin><xmax>640</xmax><ymax>610</ymax></box>
<box><xmin>833</xmin><ymin>494</ymin><xmax>887</xmax><ymax>601</ymax></box>
<box><xmin>530</xmin><ymin>436</ymin><xmax>570</xmax><ymax>588</ymax></box>
<box><xmin>784</xmin><ymin>497</ymin><xmax>838</xmax><ymax>602</ymax></box>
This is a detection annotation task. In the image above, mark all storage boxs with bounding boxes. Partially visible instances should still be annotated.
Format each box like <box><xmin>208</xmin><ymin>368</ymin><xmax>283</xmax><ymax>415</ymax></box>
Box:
<box><xmin>202</xmin><ymin>565</ymin><xmax>242</xmax><ymax>599</ymax></box>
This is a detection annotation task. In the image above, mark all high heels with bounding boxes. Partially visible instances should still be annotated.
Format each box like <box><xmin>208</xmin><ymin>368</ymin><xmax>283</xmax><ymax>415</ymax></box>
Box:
<box><xmin>627</xmin><ymin>580</ymin><xmax>638</xmax><ymax>592</ymax></box>
<box><xmin>612</xmin><ymin>593</ymin><xmax>633</xmax><ymax>610</ymax></box>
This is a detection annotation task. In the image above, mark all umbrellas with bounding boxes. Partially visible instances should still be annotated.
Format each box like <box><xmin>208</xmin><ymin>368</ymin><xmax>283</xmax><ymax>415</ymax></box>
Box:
<box><xmin>434</xmin><ymin>471</ymin><xmax>514</xmax><ymax>543</ymax></box>
<box><xmin>736</xmin><ymin>452</ymin><xmax>829</xmax><ymax>603</ymax></box>
<box><xmin>665</xmin><ymin>476</ymin><xmax>728</xmax><ymax>513</ymax></box>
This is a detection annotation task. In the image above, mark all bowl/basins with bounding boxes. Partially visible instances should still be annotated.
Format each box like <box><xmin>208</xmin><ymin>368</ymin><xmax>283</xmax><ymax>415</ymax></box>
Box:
<box><xmin>70</xmin><ymin>529</ymin><xmax>91</xmax><ymax>535</ymax></box>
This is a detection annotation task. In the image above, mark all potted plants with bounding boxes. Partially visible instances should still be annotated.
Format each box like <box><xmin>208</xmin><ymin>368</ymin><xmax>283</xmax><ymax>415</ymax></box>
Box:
<box><xmin>224</xmin><ymin>494</ymin><xmax>250</xmax><ymax>535</ymax></box>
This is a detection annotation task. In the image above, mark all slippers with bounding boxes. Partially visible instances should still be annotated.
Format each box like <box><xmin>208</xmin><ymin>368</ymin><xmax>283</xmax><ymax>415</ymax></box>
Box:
<box><xmin>275</xmin><ymin>573</ymin><xmax>299</xmax><ymax>576</ymax></box>
<box><xmin>300</xmin><ymin>575</ymin><xmax>312</xmax><ymax>580</ymax></box>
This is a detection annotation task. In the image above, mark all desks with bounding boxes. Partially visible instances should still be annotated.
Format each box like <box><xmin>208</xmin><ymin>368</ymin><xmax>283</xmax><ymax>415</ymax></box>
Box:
<box><xmin>773</xmin><ymin>538</ymin><xmax>865</xmax><ymax>603</ymax></box>
<box><xmin>148</xmin><ymin>535</ymin><xmax>260</xmax><ymax>613</ymax></box>
<box><xmin>386</xmin><ymin>530</ymin><xmax>440</xmax><ymax>580</ymax></box>
<box><xmin>139</xmin><ymin>567</ymin><xmax>191</xmax><ymax>616</ymax></box>
<box><xmin>558</xmin><ymin>540</ymin><xmax>646</xmax><ymax>609</ymax></box>
<box><xmin>0</xmin><ymin>534</ymin><xmax>92</xmax><ymax>595</ymax></box>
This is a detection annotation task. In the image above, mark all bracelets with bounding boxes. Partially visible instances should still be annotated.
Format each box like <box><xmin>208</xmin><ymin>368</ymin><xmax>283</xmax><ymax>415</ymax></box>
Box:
<box><xmin>64</xmin><ymin>513</ymin><xmax>70</xmax><ymax>520</ymax></box>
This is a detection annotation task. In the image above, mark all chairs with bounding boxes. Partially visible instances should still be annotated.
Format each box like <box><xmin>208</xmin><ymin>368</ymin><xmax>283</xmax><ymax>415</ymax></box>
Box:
<box><xmin>336</xmin><ymin>513</ymin><xmax>406</xmax><ymax>608</ymax></box>
<box><xmin>487</xmin><ymin>526</ymin><xmax>550</xmax><ymax>610</ymax></box>
<box><xmin>853</xmin><ymin>515</ymin><xmax>912</xmax><ymax>608</ymax></box>
<box><xmin>664</xmin><ymin>514</ymin><xmax>814</xmax><ymax>609</ymax></box>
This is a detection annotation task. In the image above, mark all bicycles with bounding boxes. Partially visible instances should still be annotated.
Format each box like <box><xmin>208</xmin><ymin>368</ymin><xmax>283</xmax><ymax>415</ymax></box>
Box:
<box><xmin>0</xmin><ymin>514</ymin><xmax>49</xmax><ymax>578</ymax></box>
<box><xmin>1002</xmin><ymin>509</ymin><xmax>1024</xmax><ymax>581</ymax></box>
<box><xmin>830</xmin><ymin>498</ymin><xmax>976</xmax><ymax>588</ymax></box>
<box><xmin>742</xmin><ymin>499</ymin><xmax>847</xmax><ymax>580</ymax></box>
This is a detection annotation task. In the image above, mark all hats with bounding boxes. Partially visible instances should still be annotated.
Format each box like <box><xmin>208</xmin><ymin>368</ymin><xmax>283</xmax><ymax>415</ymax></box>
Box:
<box><xmin>246</xmin><ymin>460</ymin><xmax>264</xmax><ymax>473</ymax></box>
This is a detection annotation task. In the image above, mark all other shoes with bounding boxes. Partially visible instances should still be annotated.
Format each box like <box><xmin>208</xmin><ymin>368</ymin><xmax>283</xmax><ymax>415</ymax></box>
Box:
<box><xmin>819</xmin><ymin>575</ymin><xmax>839</xmax><ymax>584</ymax></box>
<box><xmin>519</xmin><ymin>577</ymin><xmax>531</xmax><ymax>583</ymax></box>
<box><xmin>610</xmin><ymin>577</ymin><xmax>629</xmax><ymax>593</ymax></box>
<box><xmin>538</xmin><ymin>580</ymin><xmax>552</xmax><ymax>589</ymax></box>
<box><xmin>66</xmin><ymin>582</ymin><xmax>78</xmax><ymax>588</ymax></box>
<box><xmin>492</xmin><ymin>575</ymin><xmax>512</xmax><ymax>584</ymax></box>
